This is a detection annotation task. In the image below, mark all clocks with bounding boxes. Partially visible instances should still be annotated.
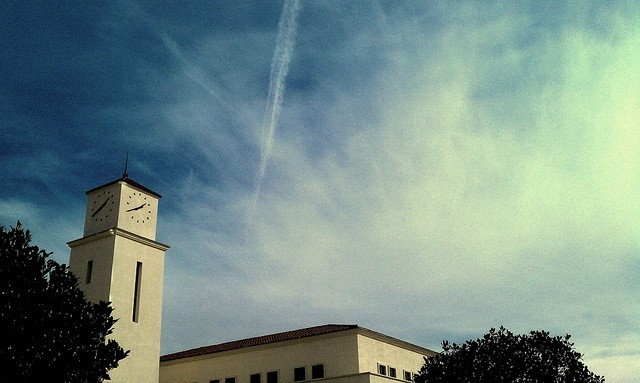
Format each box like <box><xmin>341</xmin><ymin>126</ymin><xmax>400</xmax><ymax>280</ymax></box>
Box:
<box><xmin>125</xmin><ymin>192</ymin><xmax>152</xmax><ymax>224</ymax></box>
<box><xmin>92</xmin><ymin>191</ymin><xmax>114</xmax><ymax>222</ymax></box>
